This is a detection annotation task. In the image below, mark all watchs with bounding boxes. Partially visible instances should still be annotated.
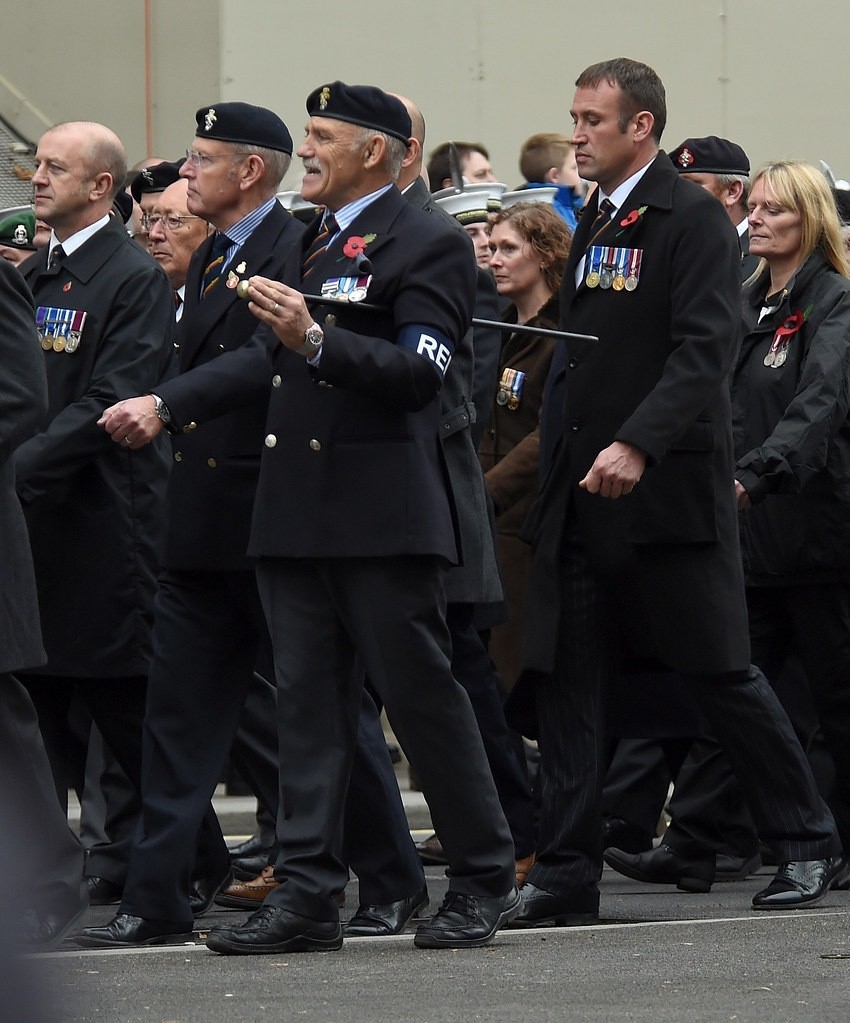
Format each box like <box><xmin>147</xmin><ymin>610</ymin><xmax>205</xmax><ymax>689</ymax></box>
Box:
<box><xmin>296</xmin><ymin>321</ymin><xmax>324</xmax><ymax>355</ymax></box>
<box><xmin>152</xmin><ymin>393</ymin><xmax>172</xmax><ymax>424</ymax></box>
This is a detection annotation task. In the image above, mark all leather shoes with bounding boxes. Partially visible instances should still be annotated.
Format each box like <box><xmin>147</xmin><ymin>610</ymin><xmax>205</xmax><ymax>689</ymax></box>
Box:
<box><xmin>0</xmin><ymin>906</ymin><xmax>86</xmax><ymax>952</ymax></box>
<box><xmin>515</xmin><ymin>849</ymin><xmax>536</xmax><ymax>889</ymax></box>
<box><xmin>750</xmin><ymin>856</ymin><xmax>850</xmax><ymax>909</ymax></box>
<box><xmin>205</xmin><ymin>904</ymin><xmax>343</xmax><ymax>954</ymax></box>
<box><xmin>413</xmin><ymin>880</ymin><xmax>525</xmax><ymax>949</ymax></box>
<box><xmin>499</xmin><ymin>880</ymin><xmax>600</xmax><ymax>930</ymax></box>
<box><xmin>189</xmin><ymin>833</ymin><xmax>280</xmax><ymax>917</ymax></box>
<box><xmin>73</xmin><ymin>911</ymin><xmax>196</xmax><ymax>948</ymax></box>
<box><xmin>413</xmin><ymin>833</ymin><xmax>450</xmax><ymax>865</ymax></box>
<box><xmin>604</xmin><ymin>842</ymin><xmax>718</xmax><ymax>893</ymax></box>
<box><xmin>86</xmin><ymin>874</ymin><xmax>124</xmax><ymax>906</ymax></box>
<box><xmin>342</xmin><ymin>883</ymin><xmax>430</xmax><ymax>937</ymax></box>
<box><xmin>715</xmin><ymin>851</ymin><xmax>763</xmax><ymax>883</ymax></box>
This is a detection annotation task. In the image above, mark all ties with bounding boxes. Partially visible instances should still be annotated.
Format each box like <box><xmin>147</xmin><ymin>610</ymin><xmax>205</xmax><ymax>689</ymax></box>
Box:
<box><xmin>200</xmin><ymin>233</ymin><xmax>236</xmax><ymax>302</ymax></box>
<box><xmin>174</xmin><ymin>291</ymin><xmax>183</xmax><ymax>311</ymax></box>
<box><xmin>300</xmin><ymin>213</ymin><xmax>341</xmax><ymax>287</ymax></box>
<box><xmin>49</xmin><ymin>244</ymin><xmax>67</xmax><ymax>270</ymax></box>
<box><xmin>584</xmin><ymin>198</ymin><xmax>617</xmax><ymax>254</ymax></box>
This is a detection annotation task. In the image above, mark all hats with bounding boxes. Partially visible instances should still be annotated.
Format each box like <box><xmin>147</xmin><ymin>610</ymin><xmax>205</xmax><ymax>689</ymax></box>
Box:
<box><xmin>429</xmin><ymin>182</ymin><xmax>560</xmax><ymax>227</ymax></box>
<box><xmin>830</xmin><ymin>186</ymin><xmax>850</xmax><ymax>226</ymax></box>
<box><xmin>195</xmin><ymin>102</ymin><xmax>293</xmax><ymax>157</ymax></box>
<box><xmin>0</xmin><ymin>210</ymin><xmax>38</xmax><ymax>251</ymax></box>
<box><xmin>668</xmin><ymin>135</ymin><xmax>751</xmax><ymax>178</ymax></box>
<box><xmin>110</xmin><ymin>157</ymin><xmax>187</xmax><ymax>225</ymax></box>
<box><xmin>306</xmin><ymin>80</ymin><xmax>412</xmax><ymax>147</ymax></box>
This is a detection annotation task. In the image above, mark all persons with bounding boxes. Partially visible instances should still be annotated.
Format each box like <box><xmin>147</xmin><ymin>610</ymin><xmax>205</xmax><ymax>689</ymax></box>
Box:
<box><xmin>0</xmin><ymin>57</ymin><xmax>850</xmax><ymax>953</ymax></box>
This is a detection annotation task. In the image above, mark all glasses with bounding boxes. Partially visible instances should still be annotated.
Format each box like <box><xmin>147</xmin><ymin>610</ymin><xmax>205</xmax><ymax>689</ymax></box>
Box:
<box><xmin>139</xmin><ymin>214</ymin><xmax>200</xmax><ymax>232</ymax></box>
<box><xmin>185</xmin><ymin>148</ymin><xmax>258</xmax><ymax>168</ymax></box>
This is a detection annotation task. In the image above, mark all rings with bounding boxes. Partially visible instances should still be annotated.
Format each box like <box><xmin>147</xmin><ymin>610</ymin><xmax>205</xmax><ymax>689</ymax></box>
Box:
<box><xmin>125</xmin><ymin>437</ymin><xmax>132</xmax><ymax>443</ymax></box>
<box><xmin>272</xmin><ymin>303</ymin><xmax>278</xmax><ymax>313</ymax></box>
